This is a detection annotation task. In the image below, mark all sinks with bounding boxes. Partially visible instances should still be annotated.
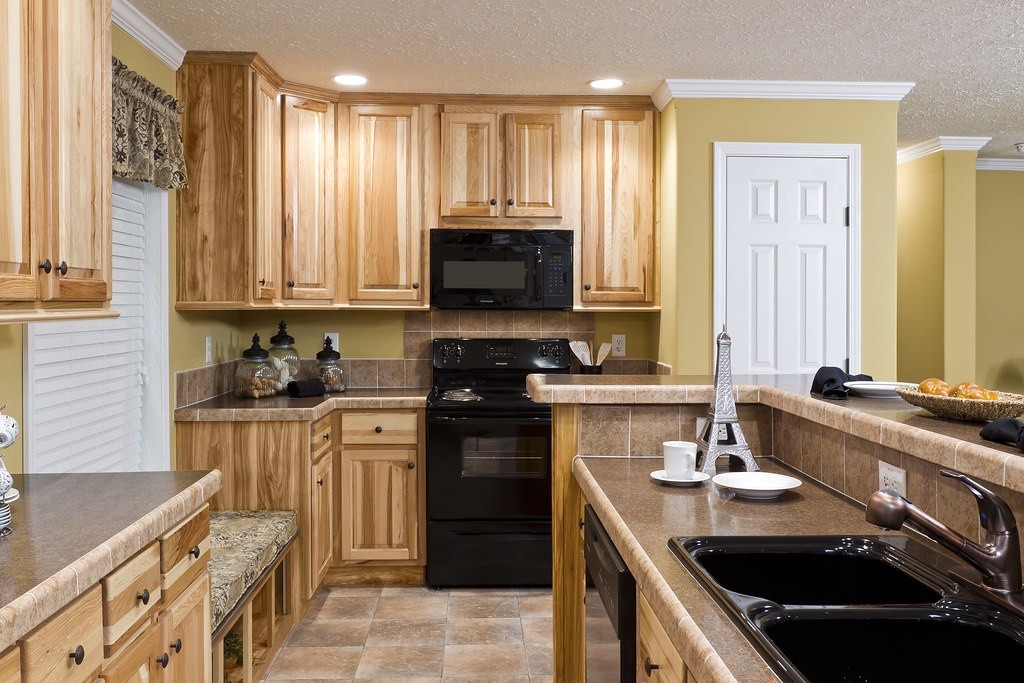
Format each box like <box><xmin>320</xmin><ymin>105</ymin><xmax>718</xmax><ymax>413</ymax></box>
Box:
<box><xmin>667</xmin><ymin>535</ymin><xmax>1024</xmax><ymax>683</ymax></box>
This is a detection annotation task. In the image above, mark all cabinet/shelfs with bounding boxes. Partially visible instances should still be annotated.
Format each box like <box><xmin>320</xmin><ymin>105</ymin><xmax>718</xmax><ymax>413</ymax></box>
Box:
<box><xmin>309</xmin><ymin>410</ymin><xmax>426</xmax><ymax>587</ymax></box>
<box><xmin>0</xmin><ymin>0</ymin><xmax>112</xmax><ymax>305</ymax></box>
<box><xmin>247</xmin><ymin>68</ymin><xmax>425</xmax><ymax>303</ymax></box>
<box><xmin>579</xmin><ymin>497</ymin><xmax>698</xmax><ymax>683</ymax></box>
<box><xmin>573</xmin><ymin>102</ymin><xmax>658</xmax><ymax>306</ymax></box>
<box><xmin>0</xmin><ymin>500</ymin><xmax>216</xmax><ymax>683</ymax></box>
<box><xmin>434</xmin><ymin>101</ymin><xmax>567</xmax><ymax>225</ymax></box>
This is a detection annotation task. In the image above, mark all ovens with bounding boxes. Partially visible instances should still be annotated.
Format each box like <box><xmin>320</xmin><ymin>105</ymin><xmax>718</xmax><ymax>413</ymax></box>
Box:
<box><xmin>424</xmin><ymin>410</ymin><xmax>551</xmax><ymax>587</ymax></box>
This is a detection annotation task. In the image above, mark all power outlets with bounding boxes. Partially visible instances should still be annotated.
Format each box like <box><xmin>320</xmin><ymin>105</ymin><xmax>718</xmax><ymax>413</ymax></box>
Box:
<box><xmin>611</xmin><ymin>333</ymin><xmax>627</xmax><ymax>357</ymax></box>
<box><xmin>694</xmin><ymin>416</ymin><xmax>729</xmax><ymax>458</ymax></box>
<box><xmin>323</xmin><ymin>332</ymin><xmax>339</xmax><ymax>351</ymax></box>
<box><xmin>206</xmin><ymin>335</ymin><xmax>213</xmax><ymax>363</ymax></box>
<box><xmin>878</xmin><ymin>460</ymin><xmax>907</xmax><ymax>498</ymax></box>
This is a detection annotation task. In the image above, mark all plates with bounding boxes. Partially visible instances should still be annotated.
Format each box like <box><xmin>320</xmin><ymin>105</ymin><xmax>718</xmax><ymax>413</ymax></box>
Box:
<box><xmin>650</xmin><ymin>469</ymin><xmax>711</xmax><ymax>486</ymax></box>
<box><xmin>844</xmin><ymin>381</ymin><xmax>919</xmax><ymax>399</ymax></box>
<box><xmin>712</xmin><ymin>471</ymin><xmax>803</xmax><ymax>500</ymax></box>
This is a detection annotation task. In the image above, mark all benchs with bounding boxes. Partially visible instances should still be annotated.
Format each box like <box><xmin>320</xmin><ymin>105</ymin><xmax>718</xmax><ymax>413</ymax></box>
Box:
<box><xmin>210</xmin><ymin>510</ymin><xmax>300</xmax><ymax>683</ymax></box>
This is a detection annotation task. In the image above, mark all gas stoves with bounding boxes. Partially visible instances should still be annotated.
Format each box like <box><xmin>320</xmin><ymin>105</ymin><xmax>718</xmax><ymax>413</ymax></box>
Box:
<box><xmin>428</xmin><ymin>338</ymin><xmax>572</xmax><ymax>410</ymax></box>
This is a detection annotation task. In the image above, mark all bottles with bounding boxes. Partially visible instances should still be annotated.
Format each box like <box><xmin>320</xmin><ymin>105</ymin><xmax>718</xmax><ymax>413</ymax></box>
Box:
<box><xmin>268</xmin><ymin>321</ymin><xmax>300</xmax><ymax>396</ymax></box>
<box><xmin>313</xmin><ymin>336</ymin><xmax>347</xmax><ymax>393</ymax></box>
<box><xmin>235</xmin><ymin>334</ymin><xmax>276</xmax><ymax>399</ymax></box>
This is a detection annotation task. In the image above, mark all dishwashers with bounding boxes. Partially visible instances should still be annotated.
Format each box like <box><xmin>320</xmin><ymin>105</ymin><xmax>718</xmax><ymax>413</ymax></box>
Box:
<box><xmin>585</xmin><ymin>504</ymin><xmax>635</xmax><ymax>683</ymax></box>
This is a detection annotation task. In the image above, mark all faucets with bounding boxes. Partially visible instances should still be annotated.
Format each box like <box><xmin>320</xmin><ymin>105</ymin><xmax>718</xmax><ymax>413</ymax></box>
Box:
<box><xmin>864</xmin><ymin>469</ymin><xmax>1024</xmax><ymax>620</ymax></box>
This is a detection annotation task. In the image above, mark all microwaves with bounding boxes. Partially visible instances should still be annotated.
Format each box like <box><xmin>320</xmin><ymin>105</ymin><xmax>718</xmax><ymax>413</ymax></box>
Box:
<box><xmin>429</xmin><ymin>228</ymin><xmax>573</xmax><ymax>312</ymax></box>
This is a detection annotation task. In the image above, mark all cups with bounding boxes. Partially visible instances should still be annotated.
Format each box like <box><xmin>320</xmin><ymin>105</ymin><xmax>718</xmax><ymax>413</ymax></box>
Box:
<box><xmin>662</xmin><ymin>441</ymin><xmax>697</xmax><ymax>480</ymax></box>
<box><xmin>580</xmin><ymin>365</ymin><xmax>602</xmax><ymax>374</ymax></box>
